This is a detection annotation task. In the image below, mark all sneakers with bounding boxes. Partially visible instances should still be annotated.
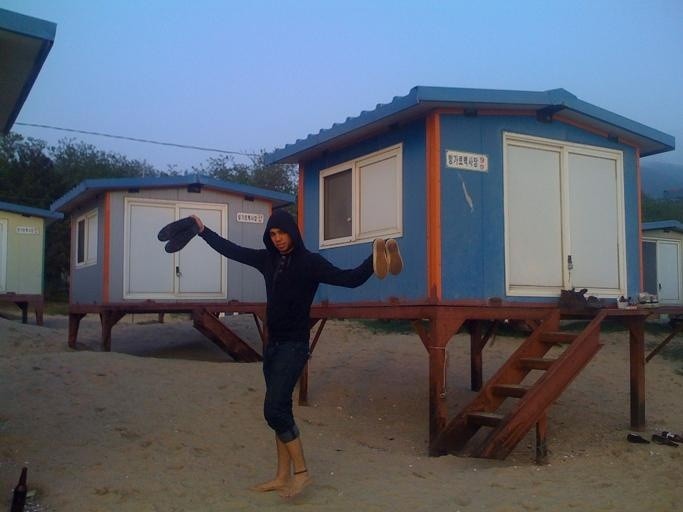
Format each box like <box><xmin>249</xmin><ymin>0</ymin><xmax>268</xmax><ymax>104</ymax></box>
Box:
<box><xmin>384</xmin><ymin>238</ymin><xmax>405</xmax><ymax>277</ymax></box>
<box><xmin>371</xmin><ymin>237</ymin><xmax>387</xmax><ymax>280</ymax></box>
<box><xmin>164</xmin><ymin>222</ymin><xmax>200</xmax><ymax>253</ymax></box>
<box><xmin>157</xmin><ymin>217</ymin><xmax>197</xmax><ymax>242</ymax></box>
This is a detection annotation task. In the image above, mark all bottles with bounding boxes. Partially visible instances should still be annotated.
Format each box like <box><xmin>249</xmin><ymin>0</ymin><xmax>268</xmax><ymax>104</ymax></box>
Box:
<box><xmin>627</xmin><ymin>433</ymin><xmax>649</xmax><ymax>444</ymax></box>
<box><xmin>652</xmin><ymin>431</ymin><xmax>683</xmax><ymax>447</ymax></box>
<box><xmin>10</xmin><ymin>467</ymin><xmax>28</xmax><ymax>512</ymax></box>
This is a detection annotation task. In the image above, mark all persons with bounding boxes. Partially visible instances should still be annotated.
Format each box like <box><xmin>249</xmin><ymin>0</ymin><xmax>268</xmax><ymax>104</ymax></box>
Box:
<box><xmin>188</xmin><ymin>206</ymin><xmax>387</xmax><ymax>500</ymax></box>
